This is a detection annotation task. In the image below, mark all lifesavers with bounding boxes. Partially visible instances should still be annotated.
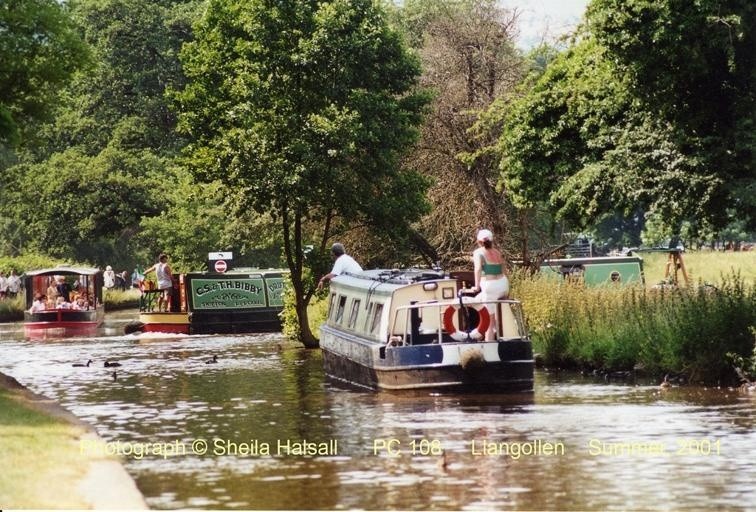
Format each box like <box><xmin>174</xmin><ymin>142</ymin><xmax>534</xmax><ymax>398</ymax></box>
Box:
<box><xmin>443</xmin><ymin>296</ymin><xmax>490</xmax><ymax>341</ymax></box>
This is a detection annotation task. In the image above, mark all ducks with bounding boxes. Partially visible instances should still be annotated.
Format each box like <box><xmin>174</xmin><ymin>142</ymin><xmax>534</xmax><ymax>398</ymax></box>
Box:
<box><xmin>205</xmin><ymin>356</ymin><xmax>218</xmax><ymax>364</ymax></box>
<box><xmin>104</xmin><ymin>362</ymin><xmax>122</xmax><ymax>367</ymax></box>
<box><xmin>660</xmin><ymin>374</ymin><xmax>671</xmax><ymax>387</ymax></box>
<box><xmin>72</xmin><ymin>360</ymin><xmax>93</xmax><ymax>367</ymax></box>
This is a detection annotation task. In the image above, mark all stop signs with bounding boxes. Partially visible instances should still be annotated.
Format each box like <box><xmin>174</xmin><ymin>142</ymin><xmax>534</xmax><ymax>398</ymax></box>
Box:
<box><xmin>214</xmin><ymin>260</ymin><xmax>227</xmax><ymax>273</ymax></box>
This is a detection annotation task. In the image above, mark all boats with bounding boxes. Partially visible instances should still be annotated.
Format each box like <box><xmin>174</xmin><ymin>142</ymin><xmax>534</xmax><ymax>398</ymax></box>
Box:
<box><xmin>319</xmin><ymin>269</ymin><xmax>536</xmax><ymax>393</ymax></box>
<box><xmin>22</xmin><ymin>265</ymin><xmax>105</xmax><ymax>342</ymax></box>
<box><xmin>124</xmin><ymin>271</ymin><xmax>291</xmax><ymax>334</ymax></box>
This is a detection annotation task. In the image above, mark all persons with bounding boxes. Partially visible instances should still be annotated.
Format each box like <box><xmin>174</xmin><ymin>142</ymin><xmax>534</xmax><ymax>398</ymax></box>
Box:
<box><xmin>0</xmin><ymin>264</ymin><xmax>144</xmax><ymax>315</ymax></box>
<box><xmin>142</xmin><ymin>254</ymin><xmax>178</xmax><ymax>313</ymax></box>
<box><xmin>318</xmin><ymin>242</ymin><xmax>363</xmax><ymax>289</ymax></box>
<box><xmin>471</xmin><ymin>229</ymin><xmax>509</xmax><ymax>342</ymax></box>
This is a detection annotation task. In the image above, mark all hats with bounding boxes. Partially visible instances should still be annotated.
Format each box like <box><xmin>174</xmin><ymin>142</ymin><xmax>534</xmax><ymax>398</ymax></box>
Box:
<box><xmin>477</xmin><ymin>229</ymin><xmax>492</xmax><ymax>242</ymax></box>
